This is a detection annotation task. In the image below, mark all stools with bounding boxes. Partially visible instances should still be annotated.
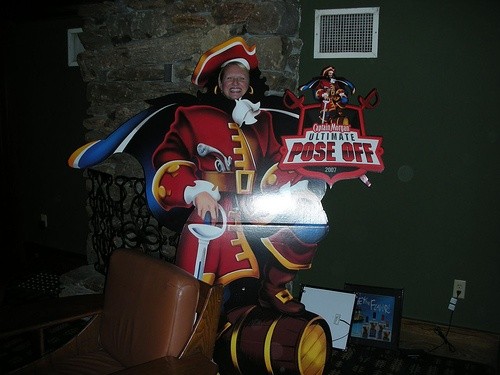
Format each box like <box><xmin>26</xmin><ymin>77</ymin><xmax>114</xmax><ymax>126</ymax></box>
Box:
<box><xmin>15</xmin><ymin>270</ymin><xmax>68</xmax><ymax>359</ymax></box>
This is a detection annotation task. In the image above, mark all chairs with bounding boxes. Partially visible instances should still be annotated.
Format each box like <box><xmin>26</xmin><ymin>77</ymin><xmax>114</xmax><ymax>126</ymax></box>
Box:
<box><xmin>11</xmin><ymin>249</ymin><xmax>226</xmax><ymax>374</ymax></box>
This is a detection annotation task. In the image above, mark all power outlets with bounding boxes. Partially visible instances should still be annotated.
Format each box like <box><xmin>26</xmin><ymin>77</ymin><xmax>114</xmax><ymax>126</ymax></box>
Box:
<box><xmin>452</xmin><ymin>279</ymin><xmax>466</xmax><ymax>299</ymax></box>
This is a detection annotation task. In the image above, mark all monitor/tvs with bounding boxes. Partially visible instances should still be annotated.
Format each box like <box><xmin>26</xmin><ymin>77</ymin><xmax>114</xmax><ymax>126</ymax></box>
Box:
<box><xmin>343</xmin><ymin>283</ymin><xmax>404</xmax><ymax>352</ymax></box>
<box><xmin>296</xmin><ymin>283</ymin><xmax>357</xmax><ymax>354</ymax></box>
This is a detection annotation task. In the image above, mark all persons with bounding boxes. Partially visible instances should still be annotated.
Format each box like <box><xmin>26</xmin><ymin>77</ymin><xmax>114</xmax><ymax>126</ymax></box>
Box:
<box><xmin>220</xmin><ymin>61</ymin><xmax>250</xmax><ymax>99</ymax></box>
